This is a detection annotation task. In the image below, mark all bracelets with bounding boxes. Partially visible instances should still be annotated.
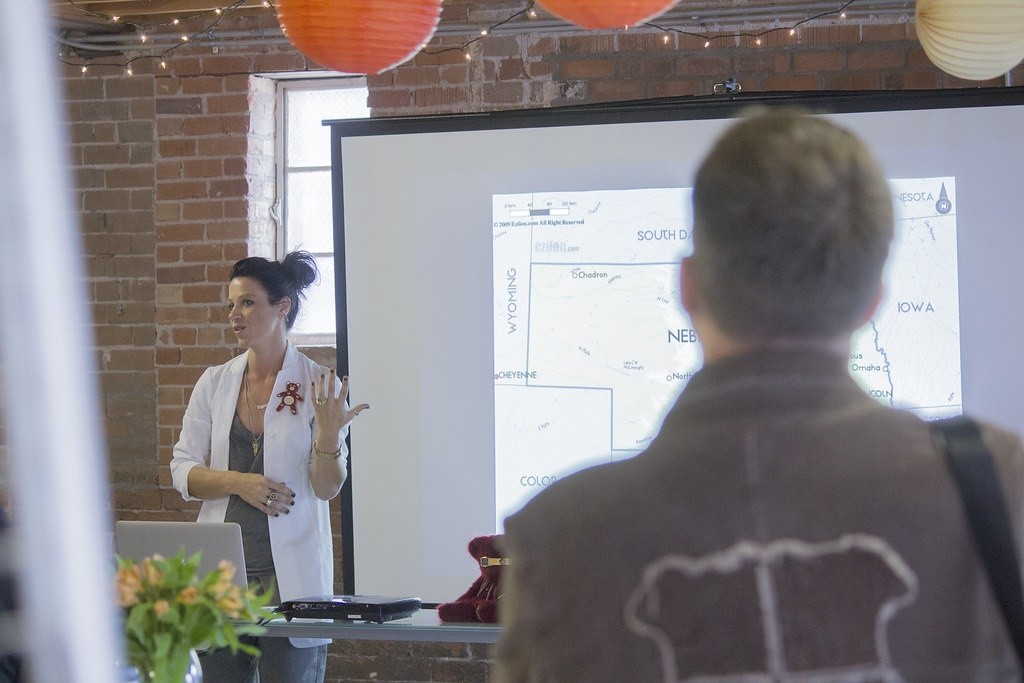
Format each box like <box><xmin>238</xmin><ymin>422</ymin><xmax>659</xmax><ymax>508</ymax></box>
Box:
<box><xmin>313</xmin><ymin>442</ymin><xmax>342</xmax><ymax>459</ymax></box>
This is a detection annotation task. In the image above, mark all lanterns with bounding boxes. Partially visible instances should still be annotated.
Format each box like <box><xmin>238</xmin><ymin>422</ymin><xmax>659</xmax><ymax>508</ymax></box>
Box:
<box><xmin>273</xmin><ymin>0</ymin><xmax>443</xmax><ymax>76</ymax></box>
<box><xmin>914</xmin><ymin>0</ymin><xmax>1024</xmax><ymax>80</ymax></box>
<box><xmin>534</xmin><ymin>0</ymin><xmax>679</xmax><ymax>29</ymax></box>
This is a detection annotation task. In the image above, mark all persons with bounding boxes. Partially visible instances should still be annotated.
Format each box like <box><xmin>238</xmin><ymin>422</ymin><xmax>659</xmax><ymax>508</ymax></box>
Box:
<box><xmin>490</xmin><ymin>106</ymin><xmax>1024</xmax><ymax>683</ymax></box>
<box><xmin>169</xmin><ymin>247</ymin><xmax>370</xmax><ymax>683</ymax></box>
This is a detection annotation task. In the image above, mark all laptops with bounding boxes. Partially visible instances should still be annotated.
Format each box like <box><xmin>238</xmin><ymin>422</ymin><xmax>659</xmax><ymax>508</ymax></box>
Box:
<box><xmin>116</xmin><ymin>520</ymin><xmax>279</xmax><ymax>628</ymax></box>
<box><xmin>281</xmin><ymin>594</ymin><xmax>422</xmax><ymax>624</ymax></box>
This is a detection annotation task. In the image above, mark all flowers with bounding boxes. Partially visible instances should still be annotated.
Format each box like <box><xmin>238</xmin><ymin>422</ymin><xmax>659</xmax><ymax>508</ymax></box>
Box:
<box><xmin>113</xmin><ymin>542</ymin><xmax>287</xmax><ymax>683</ymax></box>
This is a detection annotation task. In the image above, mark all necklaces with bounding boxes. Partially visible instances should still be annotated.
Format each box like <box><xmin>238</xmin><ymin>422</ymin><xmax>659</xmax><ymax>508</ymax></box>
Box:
<box><xmin>245</xmin><ymin>363</ymin><xmax>268</xmax><ymax>459</ymax></box>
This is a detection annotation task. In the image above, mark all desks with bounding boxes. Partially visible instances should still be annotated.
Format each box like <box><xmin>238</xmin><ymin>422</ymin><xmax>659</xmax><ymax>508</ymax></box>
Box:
<box><xmin>241</xmin><ymin>604</ymin><xmax>503</xmax><ymax>645</ymax></box>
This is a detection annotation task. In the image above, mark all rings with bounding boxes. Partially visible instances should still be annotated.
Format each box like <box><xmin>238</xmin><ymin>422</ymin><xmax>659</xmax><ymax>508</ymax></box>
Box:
<box><xmin>266</xmin><ymin>490</ymin><xmax>277</xmax><ymax>505</ymax></box>
<box><xmin>352</xmin><ymin>408</ymin><xmax>359</xmax><ymax>416</ymax></box>
<box><xmin>316</xmin><ymin>398</ymin><xmax>327</xmax><ymax>406</ymax></box>
